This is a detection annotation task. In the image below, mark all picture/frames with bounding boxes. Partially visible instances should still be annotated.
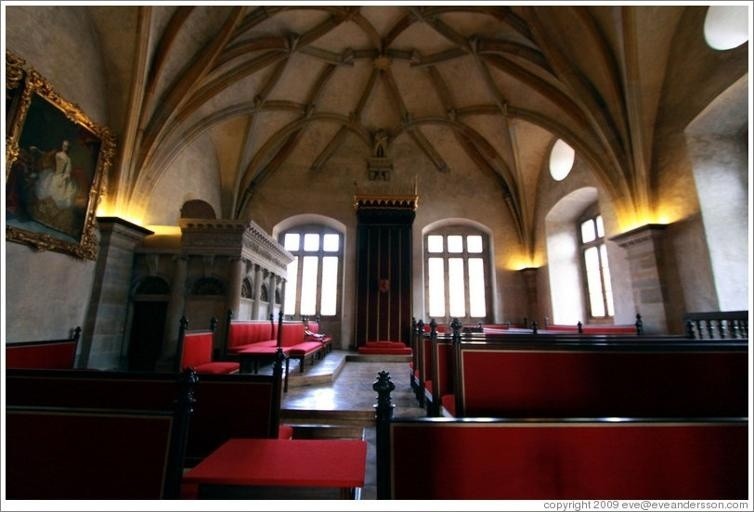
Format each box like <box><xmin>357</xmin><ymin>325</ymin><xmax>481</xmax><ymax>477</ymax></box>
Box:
<box><xmin>6</xmin><ymin>48</ymin><xmax>117</xmax><ymax>261</ymax></box>
